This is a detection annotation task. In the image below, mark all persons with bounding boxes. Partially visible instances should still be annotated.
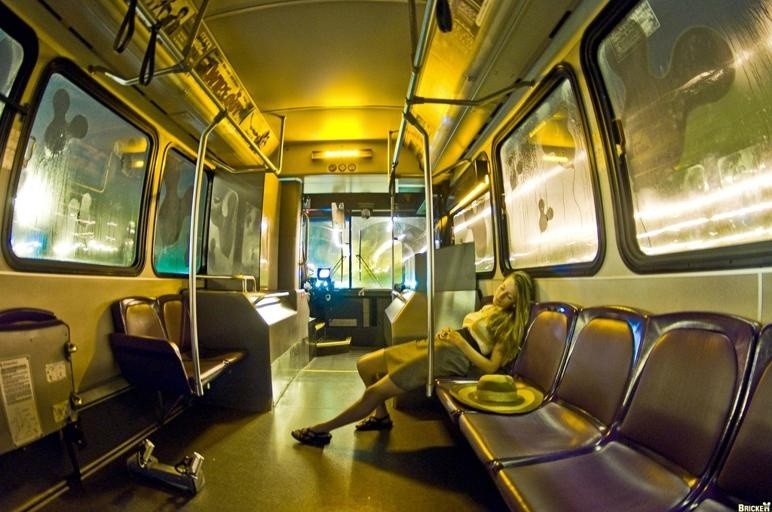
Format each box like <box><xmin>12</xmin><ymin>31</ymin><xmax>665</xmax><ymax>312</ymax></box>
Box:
<box><xmin>290</xmin><ymin>269</ymin><xmax>535</xmax><ymax>447</ymax></box>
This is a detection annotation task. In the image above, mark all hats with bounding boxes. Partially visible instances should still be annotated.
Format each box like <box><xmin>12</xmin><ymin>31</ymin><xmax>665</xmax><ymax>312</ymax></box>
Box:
<box><xmin>450</xmin><ymin>374</ymin><xmax>543</xmax><ymax>416</ymax></box>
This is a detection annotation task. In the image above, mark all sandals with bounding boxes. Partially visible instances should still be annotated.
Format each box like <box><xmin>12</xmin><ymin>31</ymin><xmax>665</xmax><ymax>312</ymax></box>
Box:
<box><xmin>288</xmin><ymin>426</ymin><xmax>332</xmax><ymax>442</ymax></box>
<box><xmin>356</xmin><ymin>415</ymin><xmax>393</xmax><ymax>431</ymax></box>
